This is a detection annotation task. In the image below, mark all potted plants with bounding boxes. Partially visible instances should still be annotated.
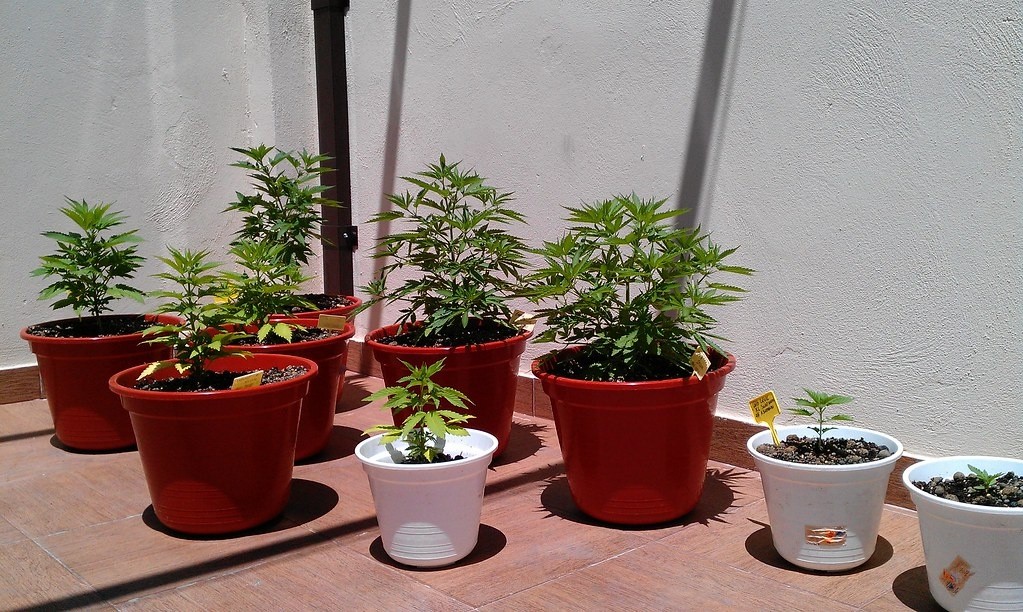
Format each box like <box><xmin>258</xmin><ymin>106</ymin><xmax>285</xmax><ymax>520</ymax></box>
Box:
<box><xmin>902</xmin><ymin>457</ymin><xmax>1023</xmax><ymax>612</ymax></box>
<box><xmin>198</xmin><ymin>205</ymin><xmax>357</xmax><ymax>469</ymax></box>
<box><xmin>525</xmin><ymin>190</ymin><xmax>752</xmax><ymax>527</ymax></box>
<box><xmin>111</xmin><ymin>248</ymin><xmax>319</xmax><ymax>532</ymax></box>
<box><xmin>233</xmin><ymin>138</ymin><xmax>365</xmax><ymax>398</ymax></box>
<box><xmin>24</xmin><ymin>195</ymin><xmax>185</xmax><ymax>456</ymax></box>
<box><xmin>747</xmin><ymin>386</ymin><xmax>904</xmax><ymax>568</ymax></box>
<box><xmin>355</xmin><ymin>359</ymin><xmax>500</xmax><ymax>565</ymax></box>
<box><xmin>365</xmin><ymin>151</ymin><xmax>534</xmax><ymax>461</ymax></box>
